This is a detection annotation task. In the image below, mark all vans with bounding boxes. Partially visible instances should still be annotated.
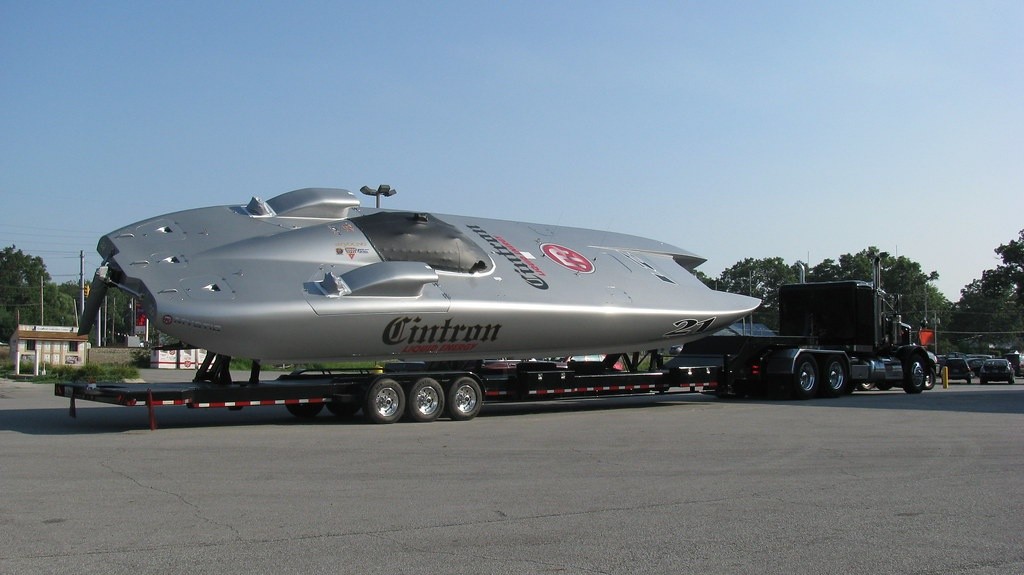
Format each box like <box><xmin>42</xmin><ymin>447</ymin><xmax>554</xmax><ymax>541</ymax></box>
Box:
<box><xmin>1002</xmin><ymin>353</ymin><xmax>1024</xmax><ymax>378</ymax></box>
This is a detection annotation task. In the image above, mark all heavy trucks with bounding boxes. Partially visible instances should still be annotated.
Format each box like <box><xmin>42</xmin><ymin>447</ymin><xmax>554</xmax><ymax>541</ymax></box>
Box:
<box><xmin>45</xmin><ymin>252</ymin><xmax>943</xmax><ymax>423</ymax></box>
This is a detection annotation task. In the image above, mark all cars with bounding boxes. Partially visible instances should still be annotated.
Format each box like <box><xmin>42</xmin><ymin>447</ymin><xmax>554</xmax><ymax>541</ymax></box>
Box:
<box><xmin>940</xmin><ymin>356</ymin><xmax>972</xmax><ymax>385</ymax></box>
<box><xmin>980</xmin><ymin>359</ymin><xmax>1016</xmax><ymax>385</ymax></box>
<box><xmin>934</xmin><ymin>351</ymin><xmax>995</xmax><ymax>378</ymax></box>
